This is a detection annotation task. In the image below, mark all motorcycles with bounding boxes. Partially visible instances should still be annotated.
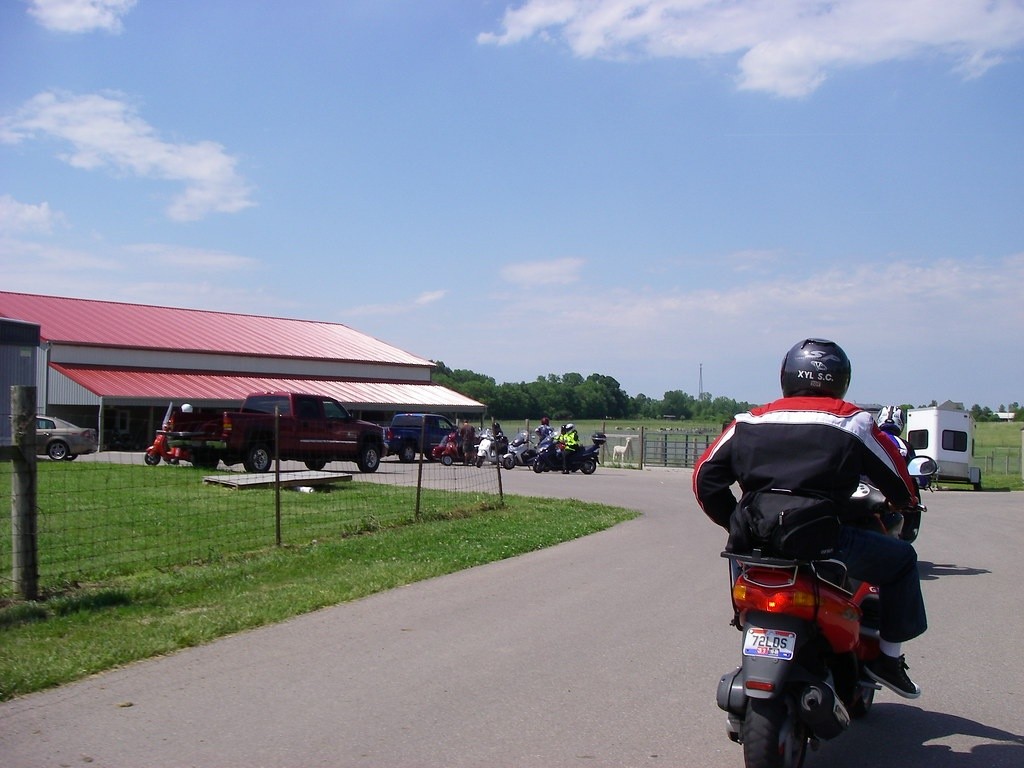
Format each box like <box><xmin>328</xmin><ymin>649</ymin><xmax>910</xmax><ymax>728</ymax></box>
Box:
<box><xmin>533</xmin><ymin>427</ymin><xmax>607</xmax><ymax>475</ymax></box>
<box><xmin>715</xmin><ymin>455</ymin><xmax>938</xmax><ymax>767</ymax></box>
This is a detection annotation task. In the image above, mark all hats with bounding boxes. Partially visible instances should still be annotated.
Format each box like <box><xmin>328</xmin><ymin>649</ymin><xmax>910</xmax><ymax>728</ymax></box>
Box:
<box><xmin>462</xmin><ymin>420</ymin><xmax>468</xmax><ymax>425</ymax></box>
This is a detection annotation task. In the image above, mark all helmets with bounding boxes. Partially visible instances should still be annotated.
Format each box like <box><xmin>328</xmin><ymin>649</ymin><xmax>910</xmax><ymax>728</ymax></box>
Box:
<box><xmin>495</xmin><ymin>422</ymin><xmax>500</xmax><ymax>429</ymax></box>
<box><xmin>780</xmin><ymin>338</ymin><xmax>852</xmax><ymax>399</ymax></box>
<box><xmin>542</xmin><ymin>417</ymin><xmax>549</xmax><ymax>425</ymax></box>
<box><xmin>876</xmin><ymin>405</ymin><xmax>905</xmax><ymax>436</ymax></box>
<box><xmin>565</xmin><ymin>424</ymin><xmax>575</xmax><ymax>434</ymax></box>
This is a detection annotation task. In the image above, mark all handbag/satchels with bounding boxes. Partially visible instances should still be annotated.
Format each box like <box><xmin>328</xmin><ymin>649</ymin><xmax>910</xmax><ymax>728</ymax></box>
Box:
<box><xmin>724</xmin><ymin>485</ymin><xmax>850</xmax><ymax>558</ymax></box>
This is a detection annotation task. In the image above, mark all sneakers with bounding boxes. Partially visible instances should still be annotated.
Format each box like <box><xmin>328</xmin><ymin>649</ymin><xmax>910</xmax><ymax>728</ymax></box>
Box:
<box><xmin>863</xmin><ymin>648</ymin><xmax>921</xmax><ymax>700</ymax></box>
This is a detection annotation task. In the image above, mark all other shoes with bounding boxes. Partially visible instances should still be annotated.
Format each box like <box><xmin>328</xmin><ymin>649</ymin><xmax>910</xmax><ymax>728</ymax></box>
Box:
<box><xmin>563</xmin><ymin>469</ymin><xmax>569</xmax><ymax>473</ymax></box>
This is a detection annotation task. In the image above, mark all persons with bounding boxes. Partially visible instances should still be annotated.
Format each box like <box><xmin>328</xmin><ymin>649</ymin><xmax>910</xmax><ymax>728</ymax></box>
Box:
<box><xmin>459</xmin><ymin>420</ymin><xmax>476</xmax><ymax>466</ymax></box>
<box><xmin>490</xmin><ymin>423</ymin><xmax>503</xmax><ymax>456</ymax></box>
<box><xmin>535</xmin><ymin>418</ymin><xmax>554</xmax><ymax>446</ymax></box>
<box><xmin>692</xmin><ymin>338</ymin><xmax>932</xmax><ymax>699</ymax></box>
<box><xmin>551</xmin><ymin>423</ymin><xmax>579</xmax><ymax>474</ymax></box>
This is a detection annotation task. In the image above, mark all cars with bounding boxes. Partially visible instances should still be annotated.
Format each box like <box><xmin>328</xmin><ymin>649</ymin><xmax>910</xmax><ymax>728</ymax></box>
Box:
<box><xmin>8</xmin><ymin>414</ymin><xmax>97</xmax><ymax>461</ymax></box>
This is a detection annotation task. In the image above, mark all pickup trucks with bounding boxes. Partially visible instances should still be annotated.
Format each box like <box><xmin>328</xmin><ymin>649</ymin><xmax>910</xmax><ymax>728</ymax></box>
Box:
<box><xmin>155</xmin><ymin>390</ymin><xmax>389</xmax><ymax>474</ymax></box>
<box><xmin>382</xmin><ymin>412</ymin><xmax>460</xmax><ymax>465</ymax></box>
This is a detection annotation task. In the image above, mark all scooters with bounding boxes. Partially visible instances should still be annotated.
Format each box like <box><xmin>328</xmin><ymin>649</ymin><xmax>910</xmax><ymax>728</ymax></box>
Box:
<box><xmin>144</xmin><ymin>402</ymin><xmax>195</xmax><ymax>466</ymax></box>
<box><xmin>101</xmin><ymin>427</ymin><xmax>143</xmax><ymax>452</ymax></box>
<box><xmin>473</xmin><ymin>427</ymin><xmax>510</xmax><ymax>469</ymax></box>
<box><xmin>502</xmin><ymin>427</ymin><xmax>540</xmax><ymax>471</ymax></box>
<box><xmin>431</xmin><ymin>425</ymin><xmax>480</xmax><ymax>467</ymax></box>
